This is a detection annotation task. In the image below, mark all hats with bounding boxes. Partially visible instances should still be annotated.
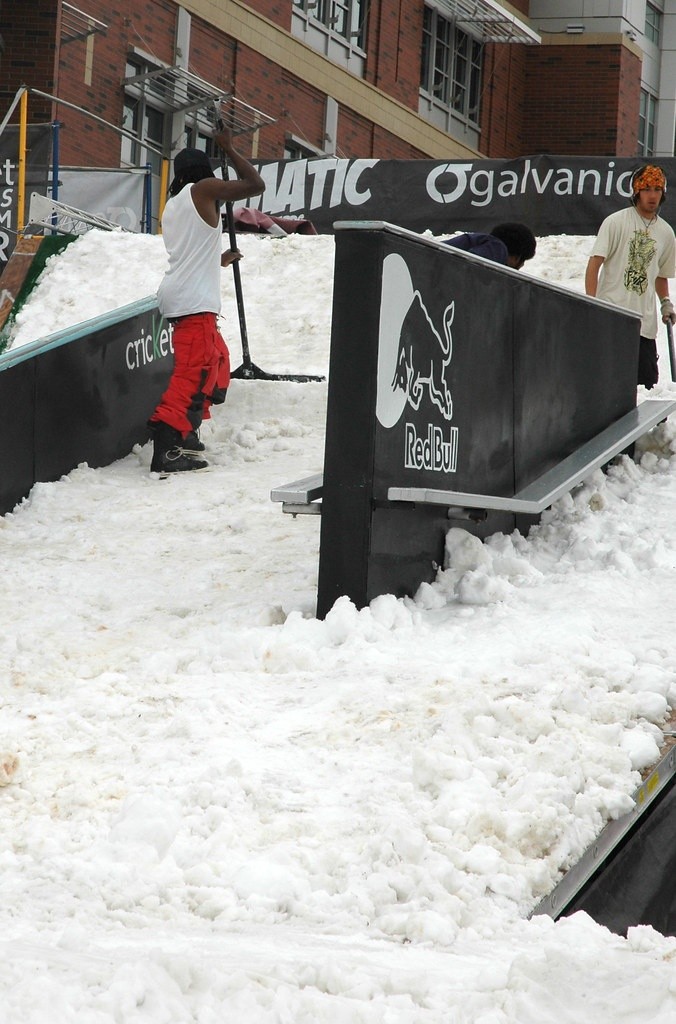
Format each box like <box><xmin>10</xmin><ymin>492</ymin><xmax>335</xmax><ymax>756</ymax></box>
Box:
<box><xmin>174</xmin><ymin>148</ymin><xmax>210</xmax><ymax>174</ymax></box>
<box><xmin>491</xmin><ymin>222</ymin><xmax>536</xmax><ymax>259</ymax></box>
<box><xmin>630</xmin><ymin>164</ymin><xmax>667</xmax><ymax>205</ymax></box>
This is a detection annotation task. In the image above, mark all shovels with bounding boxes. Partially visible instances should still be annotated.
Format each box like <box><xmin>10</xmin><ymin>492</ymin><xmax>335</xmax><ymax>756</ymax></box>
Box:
<box><xmin>214</xmin><ymin>120</ymin><xmax>328</xmax><ymax>383</ymax></box>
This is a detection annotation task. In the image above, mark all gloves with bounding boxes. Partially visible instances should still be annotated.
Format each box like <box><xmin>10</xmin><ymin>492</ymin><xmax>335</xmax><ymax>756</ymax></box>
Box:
<box><xmin>661</xmin><ymin>297</ymin><xmax>676</xmax><ymax>326</ymax></box>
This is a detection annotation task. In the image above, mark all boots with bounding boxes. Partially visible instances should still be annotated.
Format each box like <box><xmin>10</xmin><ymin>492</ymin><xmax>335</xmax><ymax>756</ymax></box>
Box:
<box><xmin>176</xmin><ymin>401</ymin><xmax>205</xmax><ymax>456</ymax></box>
<box><xmin>151</xmin><ymin>424</ymin><xmax>209</xmax><ymax>475</ymax></box>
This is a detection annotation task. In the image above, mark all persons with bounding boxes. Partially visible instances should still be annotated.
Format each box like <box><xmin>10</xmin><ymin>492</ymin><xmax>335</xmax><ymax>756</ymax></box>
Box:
<box><xmin>440</xmin><ymin>222</ymin><xmax>536</xmax><ymax>270</ymax></box>
<box><xmin>147</xmin><ymin>121</ymin><xmax>266</xmax><ymax>479</ymax></box>
<box><xmin>585</xmin><ymin>164</ymin><xmax>676</xmax><ymax>390</ymax></box>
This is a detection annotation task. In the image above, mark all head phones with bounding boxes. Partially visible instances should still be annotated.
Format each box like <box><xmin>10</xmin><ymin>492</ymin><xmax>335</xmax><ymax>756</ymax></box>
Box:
<box><xmin>629</xmin><ymin>167</ymin><xmax>667</xmax><ymax>203</ymax></box>
